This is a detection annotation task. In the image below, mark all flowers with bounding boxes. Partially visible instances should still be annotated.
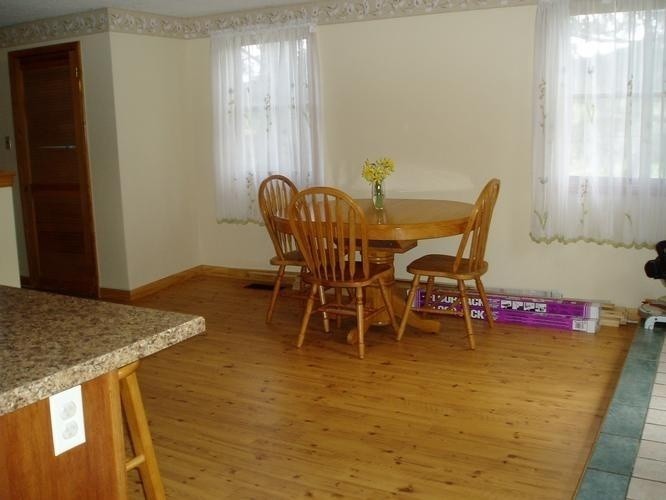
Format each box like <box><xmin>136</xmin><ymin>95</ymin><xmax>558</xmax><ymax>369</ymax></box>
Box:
<box><xmin>362</xmin><ymin>157</ymin><xmax>395</xmax><ymax>203</ymax></box>
